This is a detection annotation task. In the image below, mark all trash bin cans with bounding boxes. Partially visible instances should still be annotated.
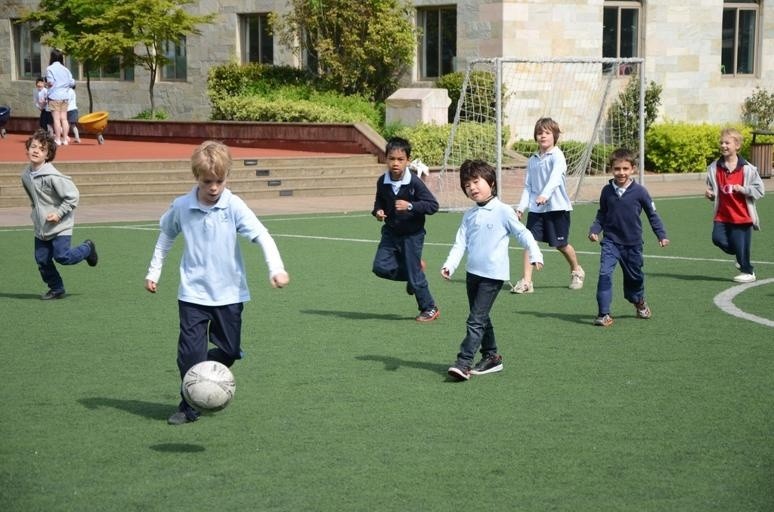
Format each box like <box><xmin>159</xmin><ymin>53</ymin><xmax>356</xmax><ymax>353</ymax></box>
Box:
<box><xmin>749</xmin><ymin>131</ymin><xmax>774</xmax><ymax>179</ymax></box>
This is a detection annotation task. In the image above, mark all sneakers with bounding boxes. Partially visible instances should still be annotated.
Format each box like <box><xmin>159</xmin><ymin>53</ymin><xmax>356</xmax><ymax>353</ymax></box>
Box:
<box><xmin>406</xmin><ymin>260</ymin><xmax>426</xmax><ymax>294</ymax></box>
<box><xmin>416</xmin><ymin>305</ymin><xmax>440</xmax><ymax>322</ymax></box>
<box><xmin>448</xmin><ymin>365</ymin><xmax>472</xmax><ymax>380</ymax></box>
<box><xmin>732</xmin><ymin>272</ymin><xmax>757</xmax><ymax>284</ymax></box>
<box><xmin>635</xmin><ymin>299</ymin><xmax>651</xmax><ymax>319</ymax></box>
<box><xmin>569</xmin><ymin>264</ymin><xmax>585</xmax><ymax>291</ymax></box>
<box><xmin>82</xmin><ymin>238</ymin><xmax>98</xmax><ymax>267</ymax></box>
<box><xmin>594</xmin><ymin>313</ymin><xmax>614</xmax><ymax>326</ymax></box>
<box><xmin>509</xmin><ymin>278</ymin><xmax>534</xmax><ymax>295</ymax></box>
<box><xmin>40</xmin><ymin>287</ymin><xmax>64</xmax><ymax>300</ymax></box>
<box><xmin>167</xmin><ymin>408</ymin><xmax>201</xmax><ymax>425</ymax></box>
<box><xmin>469</xmin><ymin>353</ymin><xmax>504</xmax><ymax>375</ymax></box>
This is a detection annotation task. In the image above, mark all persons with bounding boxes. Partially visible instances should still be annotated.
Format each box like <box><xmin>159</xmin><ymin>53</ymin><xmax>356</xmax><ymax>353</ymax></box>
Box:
<box><xmin>34</xmin><ymin>77</ymin><xmax>56</xmax><ymax>135</ymax></box>
<box><xmin>371</xmin><ymin>137</ymin><xmax>440</xmax><ymax>322</ymax></box>
<box><xmin>44</xmin><ymin>50</ymin><xmax>75</xmax><ymax>145</ymax></box>
<box><xmin>587</xmin><ymin>148</ymin><xmax>670</xmax><ymax>326</ymax></box>
<box><xmin>21</xmin><ymin>129</ymin><xmax>98</xmax><ymax>301</ymax></box>
<box><xmin>705</xmin><ymin>128</ymin><xmax>766</xmax><ymax>283</ymax></box>
<box><xmin>66</xmin><ymin>84</ymin><xmax>80</xmax><ymax>143</ymax></box>
<box><xmin>144</xmin><ymin>141</ymin><xmax>289</xmax><ymax>425</ymax></box>
<box><xmin>509</xmin><ymin>118</ymin><xmax>585</xmax><ymax>294</ymax></box>
<box><xmin>440</xmin><ymin>158</ymin><xmax>544</xmax><ymax>381</ymax></box>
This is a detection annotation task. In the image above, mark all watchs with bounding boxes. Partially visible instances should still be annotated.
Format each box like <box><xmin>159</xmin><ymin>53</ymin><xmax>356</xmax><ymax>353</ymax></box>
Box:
<box><xmin>408</xmin><ymin>203</ymin><xmax>412</xmax><ymax>211</ymax></box>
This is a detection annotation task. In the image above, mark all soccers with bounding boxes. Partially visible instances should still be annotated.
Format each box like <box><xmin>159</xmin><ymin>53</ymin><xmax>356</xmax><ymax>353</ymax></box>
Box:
<box><xmin>182</xmin><ymin>360</ymin><xmax>236</xmax><ymax>415</ymax></box>
